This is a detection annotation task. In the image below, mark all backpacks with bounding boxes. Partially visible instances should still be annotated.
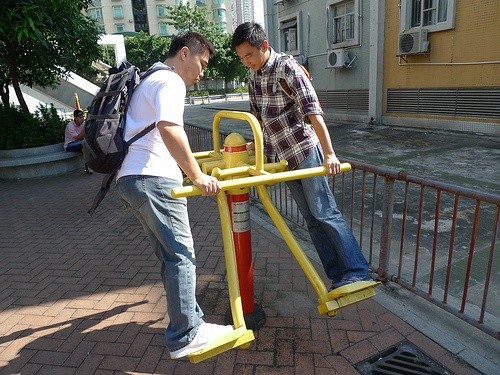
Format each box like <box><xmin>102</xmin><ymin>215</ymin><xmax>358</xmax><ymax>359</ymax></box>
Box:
<box><xmin>81</xmin><ymin>61</ymin><xmax>178</xmax><ymax>174</ymax></box>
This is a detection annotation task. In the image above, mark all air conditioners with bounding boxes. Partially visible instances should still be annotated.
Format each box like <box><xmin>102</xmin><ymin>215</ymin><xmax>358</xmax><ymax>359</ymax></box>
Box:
<box><xmin>397</xmin><ymin>29</ymin><xmax>428</xmax><ymax>56</ymax></box>
<box><xmin>326</xmin><ymin>47</ymin><xmax>349</xmax><ymax>68</ymax></box>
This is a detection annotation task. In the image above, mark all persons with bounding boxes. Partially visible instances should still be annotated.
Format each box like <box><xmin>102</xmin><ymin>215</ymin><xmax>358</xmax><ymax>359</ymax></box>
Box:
<box><xmin>115</xmin><ymin>32</ymin><xmax>234</xmax><ymax>360</ymax></box>
<box><xmin>233</xmin><ymin>22</ymin><xmax>373</xmax><ymax>293</ymax></box>
<box><xmin>64</xmin><ymin>109</ymin><xmax>86</xmax><ymax>152</ymax></box>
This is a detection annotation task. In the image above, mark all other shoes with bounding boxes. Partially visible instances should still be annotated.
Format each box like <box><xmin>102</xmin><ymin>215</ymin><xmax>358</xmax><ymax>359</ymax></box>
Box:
<box><xmin>85</xmin><ymin>168</ymin><xmax>94</xmax><ymax>173</ymax></box>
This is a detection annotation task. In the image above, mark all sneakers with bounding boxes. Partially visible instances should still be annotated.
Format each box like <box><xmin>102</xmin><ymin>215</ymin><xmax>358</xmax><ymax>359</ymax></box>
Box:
<box><xmin>170</xmin><ymin>322</ymin><xmax>234</xmax><ymax>359</ymax></box>
<box><xmin>332</xmin><ymin>275</ymin><xmax>373</xmax><ymax>289</ymax></box>
